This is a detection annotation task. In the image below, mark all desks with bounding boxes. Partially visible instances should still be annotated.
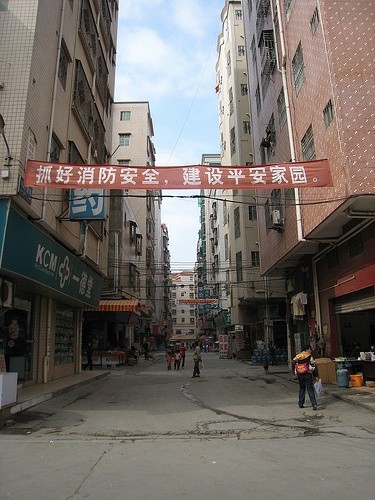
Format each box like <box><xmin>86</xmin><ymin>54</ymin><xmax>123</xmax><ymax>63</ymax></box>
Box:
<box><xmin>333</xmin><ymin>360</ymin><xmax>374</xmax><ymax>368</ymax></box>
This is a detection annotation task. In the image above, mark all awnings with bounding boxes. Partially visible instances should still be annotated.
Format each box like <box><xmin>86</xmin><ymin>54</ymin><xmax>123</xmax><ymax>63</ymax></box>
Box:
<box><xmin>82</xmin><ymin>299</ymin><xmax>142</xmax><ymax>311</ymax></box>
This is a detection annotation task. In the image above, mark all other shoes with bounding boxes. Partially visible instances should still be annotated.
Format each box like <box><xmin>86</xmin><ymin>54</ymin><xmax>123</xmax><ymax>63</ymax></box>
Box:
<box><xmin>191</xmin><ymin>375</ymin><xmax>200</xmax><ymax>378</ymax></box>
<box><xmin>313</xmin><ymin>405</ymin><xmax>318</xmax><ymax>411</ymax></box>
<box><xmin>299</xmin><ymin>405</ymin><xmax>305</xmax><ymax>408</ymax></box>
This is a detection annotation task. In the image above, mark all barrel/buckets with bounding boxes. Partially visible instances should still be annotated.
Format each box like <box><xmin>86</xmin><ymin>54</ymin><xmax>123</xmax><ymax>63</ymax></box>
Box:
<box><xmin>350</xmin><ymin>375</ymin><xmax>363</xmax><ymax>387</ymax></box>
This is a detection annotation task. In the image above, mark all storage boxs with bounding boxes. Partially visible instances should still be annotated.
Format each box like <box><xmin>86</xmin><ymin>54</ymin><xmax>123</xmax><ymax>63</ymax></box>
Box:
<box><xmin>316</xmin><ymin>359</ymin><xmax>337</xmax><ymax>385</ymax></box>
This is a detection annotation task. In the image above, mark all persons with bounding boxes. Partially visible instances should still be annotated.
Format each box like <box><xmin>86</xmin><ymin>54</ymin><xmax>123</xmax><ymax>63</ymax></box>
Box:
<box><xmin>190</xmin><ymin>342</ymin><xmax>201</xmax><ymax>378</ymax></box>
<box><xmin>82</xmin><ymin>339</ymin><xmax>95</xmax><ymax>371</ymax></box>
<box><xmin>202</xmin><ymin>343</ymin><xmax>209</xmax><ymax>353</ymax></box>
<box><xmin>292</xmin><ymin>345</ymin><xmax>319</xmax><ymax>410</ymax></box>
<box><xmin>166</xmin><ymin>342</ymin><xmax>188</xmax><ymax>370</ymax></box>
<box><xmin>143</xmin><ymin>340</ymin><xmax>149</xmax><ymax>360</ymax></box>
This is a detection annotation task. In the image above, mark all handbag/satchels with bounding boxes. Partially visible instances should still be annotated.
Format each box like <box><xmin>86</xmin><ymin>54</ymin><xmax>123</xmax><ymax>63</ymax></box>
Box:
<box><xmin>314</xmin><ymin>379</ymin><xmax>323</xmax><ymax>396</ymax></box>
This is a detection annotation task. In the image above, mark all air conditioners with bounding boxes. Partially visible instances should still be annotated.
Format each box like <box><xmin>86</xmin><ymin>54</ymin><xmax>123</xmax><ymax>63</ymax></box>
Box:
<box><xmin>270</xmin><ymin>210</ymin><xmax>281</xmax><ymax>227</ymax></box>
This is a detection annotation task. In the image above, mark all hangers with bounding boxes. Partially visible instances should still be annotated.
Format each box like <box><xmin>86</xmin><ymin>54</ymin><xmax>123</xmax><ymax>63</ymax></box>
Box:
<box><xmin>295</xmin><ymin>290</ymin><xmax>305</xmax><ymax>296</ymax></box>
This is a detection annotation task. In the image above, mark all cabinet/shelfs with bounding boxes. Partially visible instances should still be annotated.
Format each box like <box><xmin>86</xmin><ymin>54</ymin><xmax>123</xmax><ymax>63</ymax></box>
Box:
<box><xmin>229</xmin><ymin>331</ymin><xmax>252</xmax><ymax>359</ymax></box>
